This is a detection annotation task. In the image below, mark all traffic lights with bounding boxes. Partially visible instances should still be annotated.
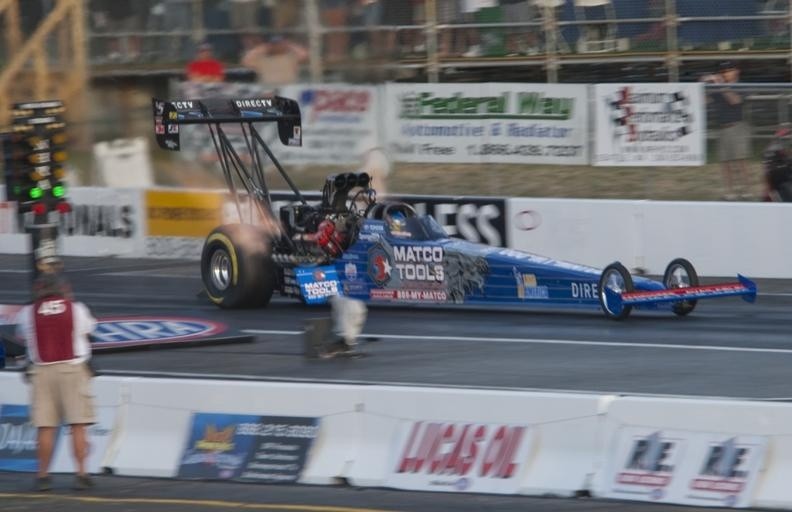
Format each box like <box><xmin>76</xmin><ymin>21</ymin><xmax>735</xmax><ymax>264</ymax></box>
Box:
<box><xmin>4</xmin><ymin>135</ymin><xmax>28</xmax><ymax>201</ymax></box>
<box><xmin>26</xmin><ymin>135</ymin><xmax>71</xmax><ymax>214</ymax></box>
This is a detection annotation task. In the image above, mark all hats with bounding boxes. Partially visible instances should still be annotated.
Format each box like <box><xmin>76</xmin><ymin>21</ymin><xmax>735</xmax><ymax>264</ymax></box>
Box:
<box><xmin>718</xmin><ymin>61</ymin><xmax>743</xmax><ymax>75</ymax></box>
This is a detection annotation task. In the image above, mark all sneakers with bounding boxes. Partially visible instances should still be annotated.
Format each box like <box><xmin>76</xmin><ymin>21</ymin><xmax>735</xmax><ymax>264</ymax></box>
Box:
<box><xmin>33</xmin><ymin>476</ymin><xmax>54</xmax><ymax>491</ymax></box>
<box><xmin>73</xmin><ymin>476</ymin><xmax>94</xmax><ymax>489</ymax></box>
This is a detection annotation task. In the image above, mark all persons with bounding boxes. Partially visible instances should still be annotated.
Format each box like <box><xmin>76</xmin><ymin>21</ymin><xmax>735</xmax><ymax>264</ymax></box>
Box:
<box><xmin>16</xmin><ymin>271</ymin><xmax>101</xmax><ymax>492</ymax></box>
<box><xmin>242</xmin><ymin>33</ymin><xmax>309</xmax><ymax>88</ymax></box>
<box><xmin>85</xmin><ymin>0</ymin><xmax>792</xmax><ymax>74</ymax></box>
<box><xmin>184</xmin><ymin>42</ymin><xmax>230</xmax><ymax>84</ymax></box>
<box><xmin>701</xmin><ymin>58</ymin><xmax>746</xmax><ymax>130</ymax></box>
